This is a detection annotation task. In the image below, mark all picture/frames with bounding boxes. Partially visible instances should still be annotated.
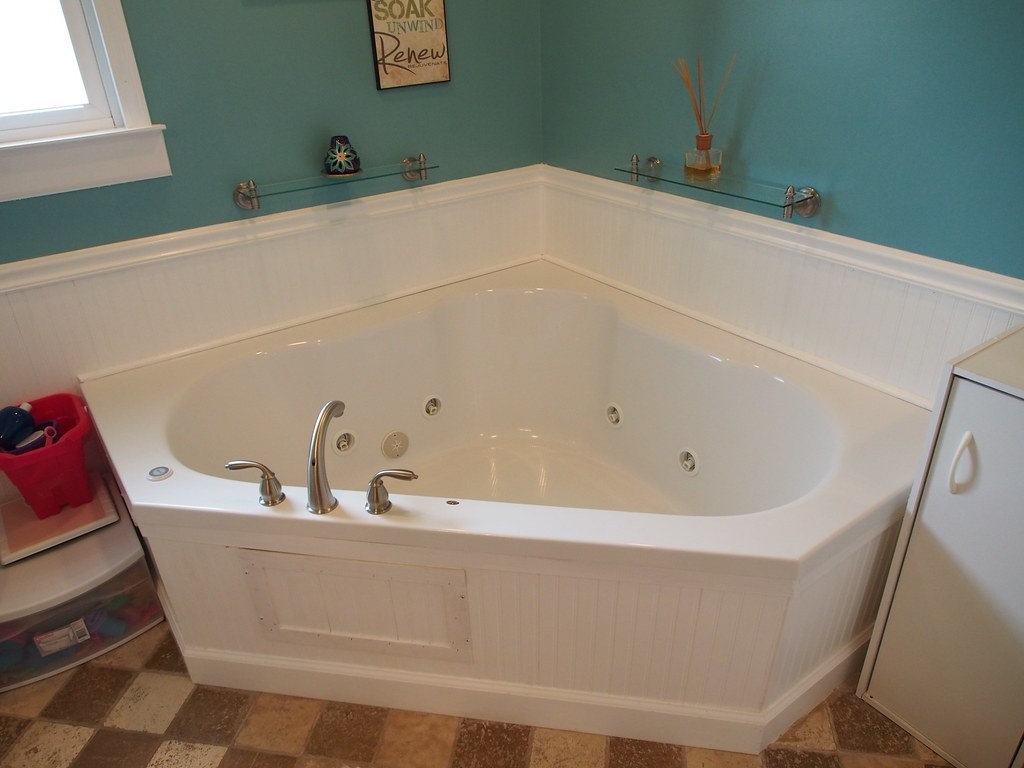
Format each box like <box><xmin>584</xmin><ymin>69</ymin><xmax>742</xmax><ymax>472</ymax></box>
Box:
<box><xmin>366</xmin><ymin>0</ymin><xmax>450</xmax><ymax>90</ymax></box>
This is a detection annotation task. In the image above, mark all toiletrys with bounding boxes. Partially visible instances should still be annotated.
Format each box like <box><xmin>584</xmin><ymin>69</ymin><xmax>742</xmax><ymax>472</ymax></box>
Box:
<box><xmin>0</xmin><ymin>401</ymin><xmax>45</xmax><ymax>452</ymax></box>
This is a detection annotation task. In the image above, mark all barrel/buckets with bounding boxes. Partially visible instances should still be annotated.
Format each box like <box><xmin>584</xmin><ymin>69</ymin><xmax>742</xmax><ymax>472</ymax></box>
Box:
<box><xmin>0</xmin><ymin>391</ymin><xmax>92</xmax><ymax>520</ymax></box>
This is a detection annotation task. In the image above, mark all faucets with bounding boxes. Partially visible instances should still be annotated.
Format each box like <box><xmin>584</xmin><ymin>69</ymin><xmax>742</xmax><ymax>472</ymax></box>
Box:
<box><xmin>306</xmin><ymin>398</ymin><xmax>347</xmax><ymax>516</ymax></box>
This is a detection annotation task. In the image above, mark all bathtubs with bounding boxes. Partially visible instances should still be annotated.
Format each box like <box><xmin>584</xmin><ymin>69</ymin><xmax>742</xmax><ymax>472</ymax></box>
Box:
<box><xmin>76</xmin><ymin>256</ymin><xmax>939</xmax><ymax>756</ymax></box>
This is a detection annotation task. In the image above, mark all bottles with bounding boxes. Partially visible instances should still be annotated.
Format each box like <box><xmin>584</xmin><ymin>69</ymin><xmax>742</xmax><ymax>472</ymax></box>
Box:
<box><xmin>0</xmin><ymin>401</ymin><xmax>34</xmax><ymax>453</ymax></box>
<box><xmin>325</xmin><ymin>135</ymin><xmax>361</xmax><ymax>175</ymax></box>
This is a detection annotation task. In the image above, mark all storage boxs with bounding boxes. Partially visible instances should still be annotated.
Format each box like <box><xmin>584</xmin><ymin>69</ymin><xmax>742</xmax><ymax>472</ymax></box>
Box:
<box><xmin>0</xmin><ymin>474</ymin><xmax>165</xmax><ymax>694</ymax></box>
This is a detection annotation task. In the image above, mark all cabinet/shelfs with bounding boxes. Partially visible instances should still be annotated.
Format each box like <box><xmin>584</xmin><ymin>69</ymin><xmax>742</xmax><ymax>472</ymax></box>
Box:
<box><xmin>855</xmin><ymin>324</ymin><xmax>1024</xmax><ymax>768</ymax></box>
